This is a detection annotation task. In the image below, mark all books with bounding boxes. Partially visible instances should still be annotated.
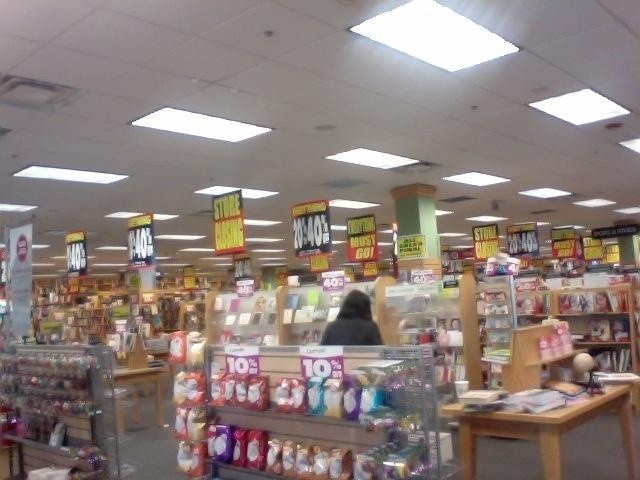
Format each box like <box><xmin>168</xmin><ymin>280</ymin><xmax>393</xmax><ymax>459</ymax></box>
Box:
<box><xmin>212</xmin><ymin>286</ymin><xmax>342</xmax><ymax>346</ymax></box>
<box><xmin>396</xmin><ymin>313</ymin><xmax>470</xmax><ymax>399</ymax></box>
<box><xmin>457</xmin><ymin>389</ymin><xmax>500</xmax><ymax>405</ymax></box>
<box><xmin>510</xmin><ymin>284</ymin><xmax>639</xmax><ymax>415</ymax></box>
<box><xmin>486</xmin><ymin>349</ymin><xmax>512</xmax><ymax>362</ymax></box>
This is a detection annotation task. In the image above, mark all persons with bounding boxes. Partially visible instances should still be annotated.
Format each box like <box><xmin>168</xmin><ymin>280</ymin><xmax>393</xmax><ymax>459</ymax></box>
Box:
<box><xmin>321</xmin><ymin>290</ymin><xmax>387</xmax><ymax>347</ymax></box>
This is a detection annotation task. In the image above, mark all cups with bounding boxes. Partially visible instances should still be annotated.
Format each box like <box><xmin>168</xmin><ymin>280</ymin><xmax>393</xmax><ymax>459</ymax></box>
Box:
<box><xmin>454</xmin><ymin>381</ymin><xmax>470</xmax><ymax>402</ymax></box>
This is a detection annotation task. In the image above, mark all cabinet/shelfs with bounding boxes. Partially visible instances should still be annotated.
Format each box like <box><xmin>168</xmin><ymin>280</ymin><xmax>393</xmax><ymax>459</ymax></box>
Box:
<box><xmin>174</xmin><ymin>342</ymin><xmax>458</xmax><ymax>480</ymax></box>
<box><xmin>175</xmin><ymin>262</ymin><xmax>639</xmax><ymax>394</ymax></box>
<box><xmin>1</xmin><ymin>272</ymin><xmax>175</xmax><ymax>478</ymax></box>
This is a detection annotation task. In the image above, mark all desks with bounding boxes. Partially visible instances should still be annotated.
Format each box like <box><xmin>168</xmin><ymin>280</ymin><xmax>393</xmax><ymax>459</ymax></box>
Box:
<box><xmin>440</xmin><ymin>375</ymin><xmax>639</xmax><ymax>479</ymax></box>
